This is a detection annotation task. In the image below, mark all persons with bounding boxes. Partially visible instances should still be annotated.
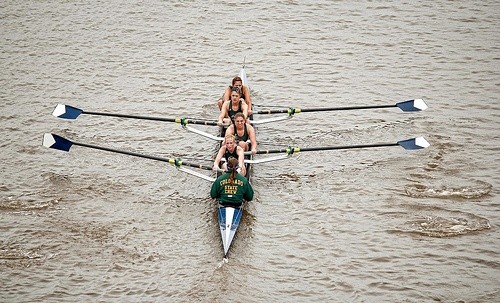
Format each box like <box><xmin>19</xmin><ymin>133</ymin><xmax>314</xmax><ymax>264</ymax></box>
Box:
<box><xmin>211</xmin><ymin>77</ymin><xmax>257</xmax><ymax>209</ymax></box>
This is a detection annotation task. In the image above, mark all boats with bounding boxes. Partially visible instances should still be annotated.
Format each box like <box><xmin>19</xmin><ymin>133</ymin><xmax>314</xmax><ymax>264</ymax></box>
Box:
<box><xmin>217</xmin><ymin>56</ymin><xmax>253</xmax><ymax>256</ymax></box>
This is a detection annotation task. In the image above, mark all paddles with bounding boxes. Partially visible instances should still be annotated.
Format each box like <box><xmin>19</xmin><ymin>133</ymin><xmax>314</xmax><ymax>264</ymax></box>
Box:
<box><xmin>243</xmin><ymin>135</ymin><xmax>431</xmax><ymax>156</ymax></box>
<box><xmin>252</xmin><ymin>97</ymin><xmax>429</xmax><ymax>115</ymax></box>
<box><xmin>50</xmin><ymin>102</ymin><xmax>229</xmax><ymax>128</ymax></box>
<box><xmin>42</xmin><ymin>131</ymin><xmax>214</xmax><ymax>171</ymax></box>
